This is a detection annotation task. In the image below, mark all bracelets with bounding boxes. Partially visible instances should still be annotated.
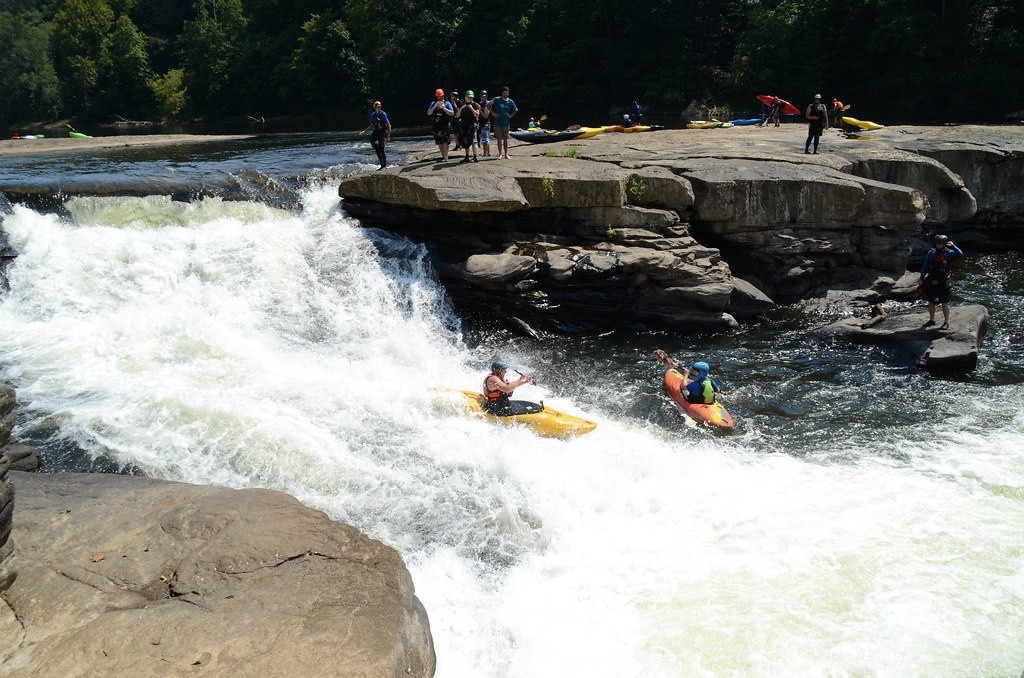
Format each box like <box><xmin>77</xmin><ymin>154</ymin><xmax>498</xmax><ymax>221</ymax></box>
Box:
<box><xmin>480</xmin><ymin>111</ymin><xmax>482</xmax><ymax>114</ymax></box>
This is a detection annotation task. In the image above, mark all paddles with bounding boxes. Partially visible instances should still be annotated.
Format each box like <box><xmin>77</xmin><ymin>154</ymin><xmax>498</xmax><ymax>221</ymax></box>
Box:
<box><xmin>655</xmin><ymin>349</ymin><xmax>732</xmax><ymax>395</ymax></box>
<box><xmin>511</xmin><ymin>367</ymin><xmax>544</xmax><ymax>389</ymax></box>
<box><xmin>829</xmin><ymin>104</ymin><xmax>850</xmax><ymax>123</ymax></box>
<box><xmin>537</xmin><ymin>114</ymin><xmax>547</xmax><ymax>123</ymax></box>
<box><xmin>712</xmin><ymin>118</ymin><xmax>720</xmax><ymax>122</ymax></box>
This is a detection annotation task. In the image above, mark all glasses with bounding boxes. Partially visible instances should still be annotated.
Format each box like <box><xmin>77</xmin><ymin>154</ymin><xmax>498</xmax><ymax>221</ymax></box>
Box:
<box><xmin>502</xmin><ymin>369</ymin><xmax>507</xmax><ymax>373</ymax></box>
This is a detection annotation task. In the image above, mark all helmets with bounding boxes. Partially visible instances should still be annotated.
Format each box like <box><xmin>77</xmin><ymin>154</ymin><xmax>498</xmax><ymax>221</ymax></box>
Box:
<box><xmin>688</xmin><ymin>362</ymin><xmax>709</xmax><ymax>380</ymax></box>
<box><xmin>466</xmin><ymin>90</ymin><xmax>474</xmax><ymax>98</ymax></box>
<box><xmin>632</xmin><ymin>100</ymin><xmax>637</xmax><ymax>104</ymax></box>
<box><xmin>530</xmin><ymin>118</ymin><xmax>534</xmax><ymax>121</ymax></box>
<box><xmin>833</xmin><ymin>98</ymin><xmax>837</xmax><ymax>102</ymax></box>
<box><xmin>436</xmin><ymin>89</ymin><xmax>444</xmax><ymax>96</ymax></box>
<box><xmin>491</xmin><ymin>361</ymin><xmax>510</xmax><ymax>371</ymax></box>
<box><xmin>480</xmin><ymin>90</ymin><xmax>487</xmax><ymax>96</ymax></box>
<box><xmin>934</xmin><ymin>235</ymin><xmax>948</xmax><ymax>255</ymax></box>
<box><xmin>815</xmin><ymin>94</ymin><xmax>822</xmax><ymax>98</ymax></box>
<box><xmin>623</xmin><ymin>114</ymin><xmax>629</xmax><ymax>120</ymax></box>
<box><xmin>374</xmin><ymin>101</ymin><xmax>381</xmax><ymax>106</ymax></box>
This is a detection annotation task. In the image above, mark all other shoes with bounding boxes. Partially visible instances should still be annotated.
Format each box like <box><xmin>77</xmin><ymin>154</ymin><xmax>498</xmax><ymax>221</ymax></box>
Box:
<box><xmin>481</xmin><ymin>154</ymin><xmax>492</xmax><ymax>157</ymax></box>
<box><xmin>923</xmin><ymin>320</ymin><xmax>935</xmax><ymax>327</ymax></box>
<box><xmin>498</xmin><ymin>155</ymin><xmax>511</xmax><ymax>160</ymax></box>
<box><xmin>452</xmin><ymin>146</ymin><xmax>461</xmax><ymax>150</ymax></box>
<box><xmin>442</xmin><ymin>156</ymin><xmax>479</xmax><ymax>163</ymax></box>
<box><xmin>805</xmin><ymin>151</ymin><xmax>818</xmax><ymax>154</ymax></box>
<box><xmin>940</xmin><ymin>323</ymin><xmax>949</xmax><ymax>330</ymax></box>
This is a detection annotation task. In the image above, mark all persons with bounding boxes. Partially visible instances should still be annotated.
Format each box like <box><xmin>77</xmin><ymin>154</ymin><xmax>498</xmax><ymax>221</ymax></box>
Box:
<box><xmin>681</xmin><ymin>362</ymin><xmax>720</xmax><ymax>403</ymax></box>
<box><xmin>805</xmin><ymin>94</ymin><xmax>828</xmax><ymax>154</ymax></box>
<box><xmin>771</xmin><ymin>97</ymin><xmax>784</xmax><ymax>127</ymax></box>
<box><xmin>257</xmin><ymin>116</ymin><xmax>266</xmax><ymax>123</ymax></box>
<box><xmin>360</xmin><ymin>101</ymin><xmax>391</xmax><ymax>171</ymax></box>
<box><xmin>621</xmin><ymin>99</ymin><xmax>642</xmax><ymax>128</ymax></box>
<box><xmin>450</xmin><ymin>90</ymin><xmax>492</xmax><ymax>162</ymax></box>
<box><xmin>917</xmin><ymin>234</ymin><xmax>963</xmax><ymax>329</ymax></box>
<box><xmin>428</xmin><ymin>89</ymin><xmax>454</xmax><ymax>162</ymax></box>
<box><xmin>529</xmin><ymin>117</ymin><xmax>541</xmax><ymax>129</ymax></box>
<box><xmin>486</xmin><ymin>87</ymin><xmax>518</xmax><ymax>160</ymax></box>
<box><xmin>833</xmin><ymin>98</ymin><xmax>843</xmax><ymax>126</ymax></box>
<box><xmin>483</xmin><ymin>361</ymin><xmax>536</xmax><ymax>408</ymax></box>
